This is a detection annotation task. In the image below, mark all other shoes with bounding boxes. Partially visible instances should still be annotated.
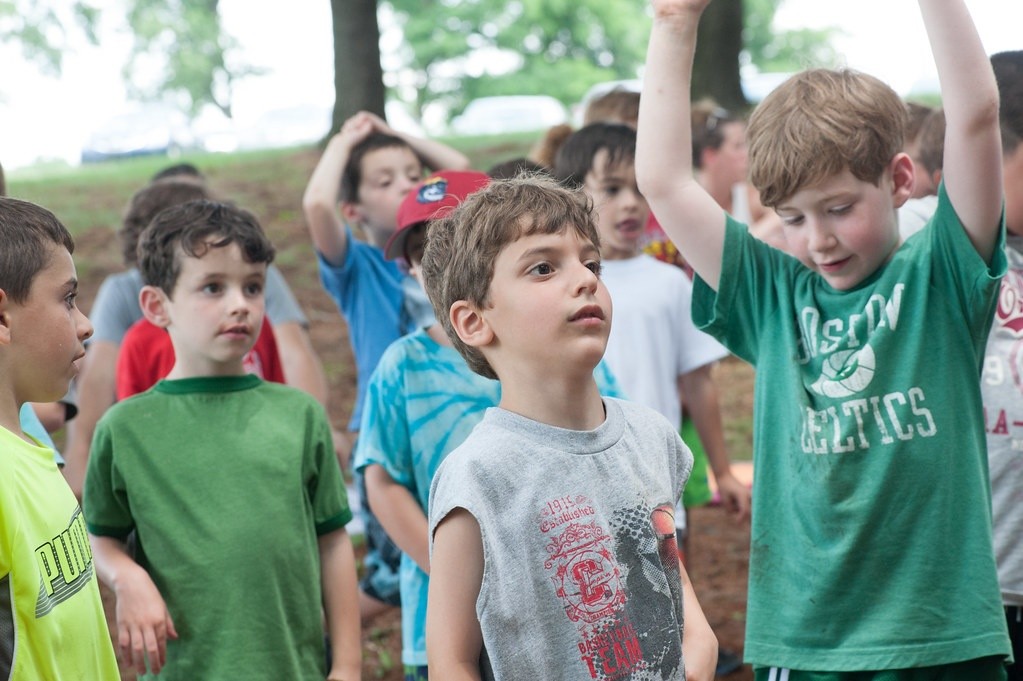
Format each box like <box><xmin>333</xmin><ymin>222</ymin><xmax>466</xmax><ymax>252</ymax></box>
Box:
<box><xmin>715</xmin><ymin>648</ymin><xmax>741</xmax><ymax>675</ymax></box>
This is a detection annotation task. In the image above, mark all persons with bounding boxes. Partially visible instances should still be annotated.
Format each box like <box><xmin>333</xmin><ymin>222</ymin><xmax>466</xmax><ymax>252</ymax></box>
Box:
<box><xmin>487</xmin><ymin>83</ymin><xmax>752</xmax><ymax>568</ymax></box>
<box><xmin>633</xmin><ymin>0</ymin><xmax>1015</xmax><ymax>681</ymax></box>
<box><xmin>302</xmin><ymin>111</ymin><xmax>470</xmax><ymax>624</ymax></box>
<box><xmin>900</xmin><ymin>51</ymin><xmax>1023</xmax><ymax>681</ymax></box>
<box><xmin>64</xmin><ymin>163</ymin><xmax>328</xmax><ymax>501</ymax></box>
<box><xmin>420</xmin><ymin>178</ymin><xmax>719</xmax><ymax>681</ymax></box>
<box><xmin>0</xmin><ymin>194</ymin><xmax>122</xmax><ymax>681</ymax></box>
<box><xmin>354</xmin><ymin>170</ymin><xmax>621</xmax><ymax>679</ymax></box>
<box><xmin>81</xmin><ymin>199</ymin><xmax>361</xmax><ymax>681</ymax></box>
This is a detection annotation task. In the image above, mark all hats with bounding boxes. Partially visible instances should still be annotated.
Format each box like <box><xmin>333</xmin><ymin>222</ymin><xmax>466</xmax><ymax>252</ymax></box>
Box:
<box><xmin>388</xmin><ymin>170</ymin><xmax>493</xmax><ymax>261</ymax></box>
